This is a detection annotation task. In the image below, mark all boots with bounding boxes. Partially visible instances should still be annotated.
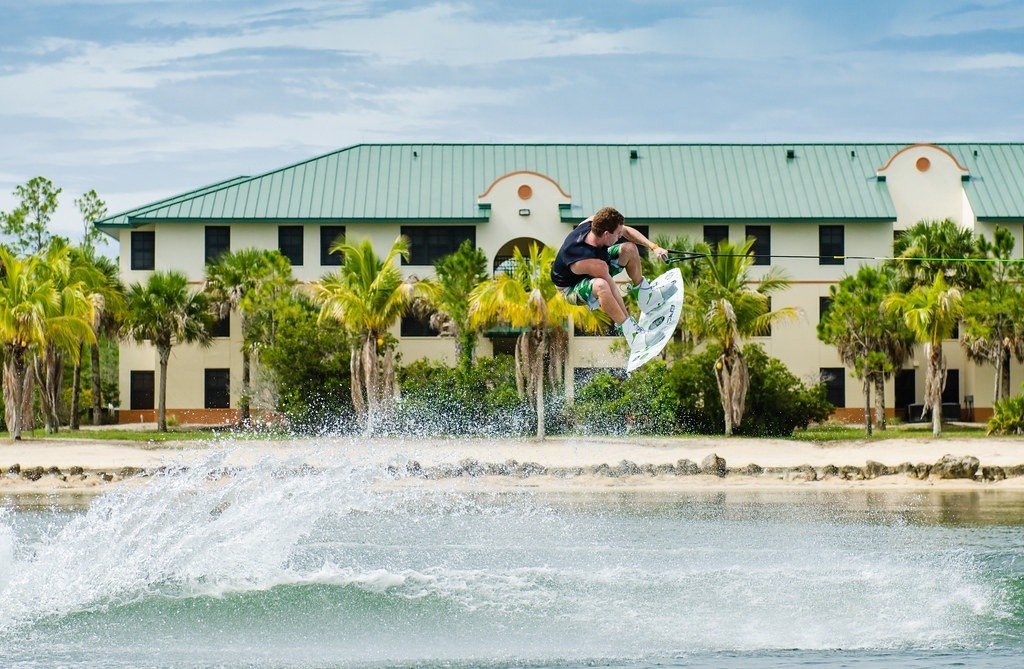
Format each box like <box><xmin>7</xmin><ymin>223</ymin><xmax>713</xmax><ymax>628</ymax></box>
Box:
<box><xmin>617</xmin><ymin>315</ymin><xmax>665</xmax><ymax>353</ymax></box>
<box><xmin>626</xmin><ymin>275</ymin><xmax>678</xmax><ymax>315</ymax></box>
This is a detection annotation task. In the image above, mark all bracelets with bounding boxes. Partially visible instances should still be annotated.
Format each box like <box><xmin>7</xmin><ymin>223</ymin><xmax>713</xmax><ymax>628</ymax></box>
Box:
<box><xmin>651</xmin><ymin>244</ymin><xmax>657</xmax><ymax>251</ymax></box>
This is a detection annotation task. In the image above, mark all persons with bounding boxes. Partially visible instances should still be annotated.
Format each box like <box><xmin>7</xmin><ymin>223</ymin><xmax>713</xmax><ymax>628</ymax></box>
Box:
<box><xmin>550</xmin><ymin>207</ymin><xmax>675</xmax><ymax>353</ymax></box>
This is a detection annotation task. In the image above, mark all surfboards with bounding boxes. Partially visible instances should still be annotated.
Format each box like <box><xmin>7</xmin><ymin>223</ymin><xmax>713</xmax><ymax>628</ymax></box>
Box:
<box><xmin>627</xmin><ymin>268</ymin><xmax>684</xmax><ymax>371</ymax></box>
